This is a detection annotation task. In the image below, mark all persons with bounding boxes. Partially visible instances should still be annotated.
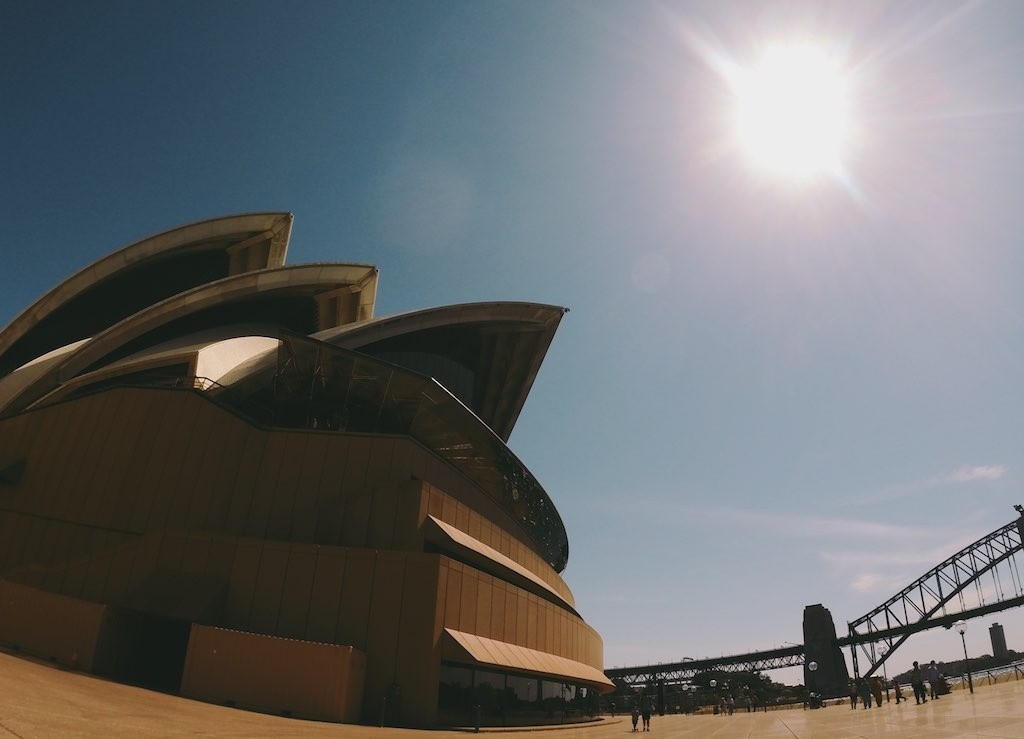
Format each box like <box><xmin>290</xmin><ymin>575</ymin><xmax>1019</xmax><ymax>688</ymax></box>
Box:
<box><xmin>911</xmin><ymin>661</ymin><xmax>928</xmax><ymax>706</ymax></box>
<box><xmin>641</xmin><ymin>692</ymin><xmax>650</xmax><ymax>732</ymax></box>
<box><xmin>811</xmin><ymin>691</ymin><xmax>823</xmax><ymax>708</ymax></box>
<box><xmin>652</xmin><ymin>704</ymin><xmax>699</xmax><ymax>717</ymax></box>
<box><xmin>925</xmin><ymin>661</ymin><xmax>943</xmax><ymax>699</ymax></box>
<box><xmin>631</xmin><ymin>704</ymin><xmax>639</xmax><ymax>732</ymax></box>
<box><xmin>894</xmin><ymin>681</ymin><xmax>907</xmax><ymax>703</ymax></box>
<box><xmin>848</xmin><ymin>679</ymin><xmax>883</xmax><ymax>708</ymax></box>
<box><xmin>612</xmin><ymin>701</ymin><xmax>615</xmax><ymax>717</ymax></box>
<box><xmin>544</xmin><ymin>699</ymin><xmax>593</xmax><ymax>721</ymax></box>
<box><xmin>720</xmin><ymin>696</ymin><xmax>734</xmax><ymax>715</ymax></box>
<box><xmin>744</xmin><ymin>693</ymin><xmax>757</xmax><ymax>712</ymax></box>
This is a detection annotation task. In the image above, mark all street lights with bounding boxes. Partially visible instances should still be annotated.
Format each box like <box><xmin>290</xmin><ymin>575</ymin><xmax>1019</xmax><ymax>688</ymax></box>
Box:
<box><xmin>808</xmin><ymin>661</ymin><xmax>818</xmax><ymax>697</ymax></box>
<box><xmin>690</xmin><ymin>685</ymin><xmax>697</xmax><ymax>712</ymax></box>
<box><xmin>709</xmin><ymin>679</ymin><xmax>717</xmax><ymax>715</ymax></box>
<box><xmin>955</xmin><ymin>619</ymin><xmax>974</xmax><ymax>694</ymax></box>
<box><xmin>876</xmin><ymin>645</ymin><xmax>890</xmax><ymax>702</ymax></box>
<box><xmin>682</xmin><ymin>684</ymin><xmax>689</xmax><ymax>715</ymax></box>
